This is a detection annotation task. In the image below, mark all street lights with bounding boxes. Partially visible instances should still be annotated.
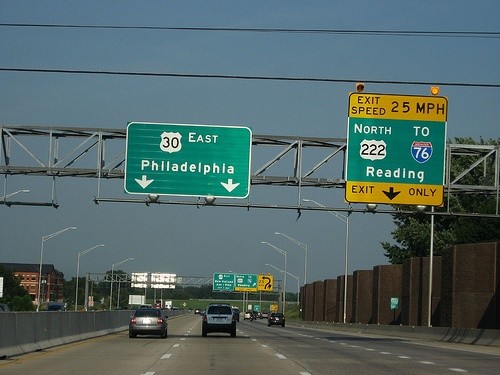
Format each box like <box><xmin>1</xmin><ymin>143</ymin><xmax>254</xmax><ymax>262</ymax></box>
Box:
<box><xmin>110</xmin><ymin>258</ymin><xmax>134</xmax><ymax>311</ymax></box>
<box><xmin>37</xmin><ymin>227</ymin><xmax>77</xmax><ymax>310</ymax></box>
<box><xmin>261</xmin><ymin>242</ymin><xmax>287</xmax><ymax>316</ymax></box>
<box><xmin>303</xmin><ymin>199</ymin><xmax>348</xmax><ymax>323</ymax></box>
<box><xmin>275</xmin><ymin>232</ymin><xmax>307</xmax><ymax>284</ymax></box>
<box><xmin>265</xmin><ymin>264</ymin><xmax>300</xmax><ymax>305</ymax></box>
<box><xmin>75</xmin><ymin>244</ymin><xmax>104</xmax><ymax>311</ymax></box>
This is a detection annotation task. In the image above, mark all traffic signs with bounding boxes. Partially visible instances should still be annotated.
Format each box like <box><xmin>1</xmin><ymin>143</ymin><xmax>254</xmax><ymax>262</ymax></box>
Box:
<box><xmin>212</xmin><ymin>272</ymin><xmax>234</xmax><ymax>292</ymax></box>
<box><xmin>345</xmin><ymin>82</ymin><xmax>448</xmax><ymax>206</ymax></box>
<box><xmin>258</xmin><ymin>275</ymin><xmax>273</xmax><ymax>291</ymax></box>
<box><xmin>124</xmin><ymin>121</ymin><xmax>253</xmax><ymax>199</ymax></box>
<box><xmin>234</xmin><ymin>273</ymin><xmax>258</xmax><ymax>292</ymax></box>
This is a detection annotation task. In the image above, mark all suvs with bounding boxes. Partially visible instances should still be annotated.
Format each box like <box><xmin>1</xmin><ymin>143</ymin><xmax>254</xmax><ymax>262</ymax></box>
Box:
<box><xmin>202</xmin><ymin>303</ymin><xmax>236</xmax><ymax>337</ymax></box>
<box><xmin>0</xmin><ymin>302</ymin><xmax>72</xmax><ymax>312</ymax></box>
<box><xmin>115</xmin><ymin>304</ymin><xmax>179</xmax><ymax>310</ymax></box>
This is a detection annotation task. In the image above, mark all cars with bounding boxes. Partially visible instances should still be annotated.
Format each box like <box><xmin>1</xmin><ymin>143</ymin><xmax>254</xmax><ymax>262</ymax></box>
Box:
<box><xmin>194</xmin><ymin>307</ymin><xmax>269</xmax><ymax>322</ymax></box>
<box><xmin>268</xmin><ymin>313</ymin><xmax>285</xmax><ymax>327</ymax></box>
<box><xmin>129</xmin><ymin>308</ymin><xmax>168</xmax><ymax>339</ymax></box>
